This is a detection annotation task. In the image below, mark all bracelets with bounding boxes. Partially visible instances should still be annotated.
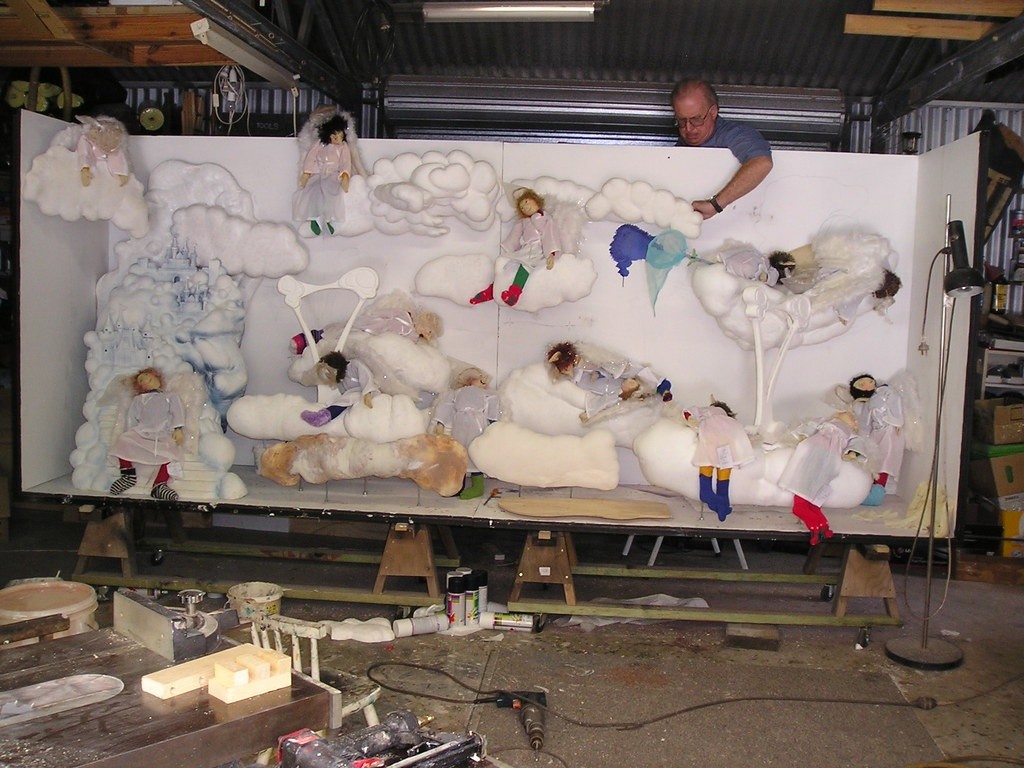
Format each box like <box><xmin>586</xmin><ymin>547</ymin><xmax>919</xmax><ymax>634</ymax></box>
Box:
<box><xmin>708</xmin><ymin>196</ymin><xmax>723</xmax><ymax>213</ymax></box>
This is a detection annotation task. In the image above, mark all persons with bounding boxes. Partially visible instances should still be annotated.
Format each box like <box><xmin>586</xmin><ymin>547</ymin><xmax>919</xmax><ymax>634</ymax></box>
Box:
<box><xmin>469</xmin><ymin>188</ymin><xmax>561</xmax><ymax>307</ymax></box>
<box><xmin>545</xmin><ymin>338</ymin><xmax>673</xmax><ymax>423</ymax></box>
<box><xmin>301</xmin><ymin>351</ymin><xmax>381</xmax><ymax>428</ymax></box>
<box><xmin>431</xmin><ymin>365</ymin><xmax>500</xmax><ymax>499</ymax></box>
<box><xmin>718</xmin><ymin>216</ymin><xmax>902</xmax><ymax>325</ymax></box>
<box><xmin>288</xmin><ymin>292</ymin><xmax>443</xmax><ymax>355</ymax></box>
<box><xmin>682</xmin><ymin>393</ymin><xmax>754</xmax><ymax>522</ymax></box>
<box><xmin>778</xmin><ymin>372</ymin><xmax>906</xmax><ymax>545</ymax></box>
<box><xmin>291</xmin><ymin>112</ymin><xmax>351</xmax><ymax>235</ymax></box>
<box><xmin>76</xmin><ymin>121</ymin><xmax>130</xmax><ymax>188</ymax></box>
<box><xmin>672</xmin><ymin>78</ymin><xmax>773</xmax><ymax>220</ymax></box>
<box><xmin>109</xmin><ymin>367</ymin><xmax>185</xmax><ymax>501</ymax></box>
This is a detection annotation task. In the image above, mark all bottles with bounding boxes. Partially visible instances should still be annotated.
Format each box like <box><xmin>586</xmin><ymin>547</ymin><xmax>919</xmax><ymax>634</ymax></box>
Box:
<box><xmin>991</xmin><ymin>268</ymin><xmax>1008</xmax><ymax>314</ymax></box>
<box><xmin>117</xmin><ymin>587</ymin><xmax>168</xmax><ymax>599</ymax></box>
<box><xmin>1014</xmin><ymin>238</ymin><xmax>1024</xmax><ymax>264</ymax></box>
<box><xmin>1013</xmin><ymin>211</ymin><xmax>1024</xmax><ymax>236</ymax></box>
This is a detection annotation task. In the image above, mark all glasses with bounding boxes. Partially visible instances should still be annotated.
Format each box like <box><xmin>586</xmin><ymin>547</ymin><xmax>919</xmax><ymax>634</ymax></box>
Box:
<box><xmin>671</xmin><ymin>104</ymin><xmax>714</xmax><ymax>128</ymax></box>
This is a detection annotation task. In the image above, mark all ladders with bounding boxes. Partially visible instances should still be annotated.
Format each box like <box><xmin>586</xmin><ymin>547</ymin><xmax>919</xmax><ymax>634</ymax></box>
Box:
<box><xmin>626</xmin><ymin>532</ymin><xmax>751</xmax><ymax>575</ymax></box>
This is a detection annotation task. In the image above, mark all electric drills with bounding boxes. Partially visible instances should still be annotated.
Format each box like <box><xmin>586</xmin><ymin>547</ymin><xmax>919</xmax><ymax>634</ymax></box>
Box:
<box><xmin>491</xmin><ymin>686</ymin><xmax>550</xmax><ymax>757</ymax></box>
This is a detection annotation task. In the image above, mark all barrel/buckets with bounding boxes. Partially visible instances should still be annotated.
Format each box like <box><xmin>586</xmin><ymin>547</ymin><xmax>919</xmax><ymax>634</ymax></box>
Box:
<box><xmin>226</xmin><ymin>582</ymin><xmax>283</xmax><ymax>623</ymax></box>
<box><xmin>0</xmin><ymin>582</ymin><xmax>99</xmax><ymax>649</ymax></box>
